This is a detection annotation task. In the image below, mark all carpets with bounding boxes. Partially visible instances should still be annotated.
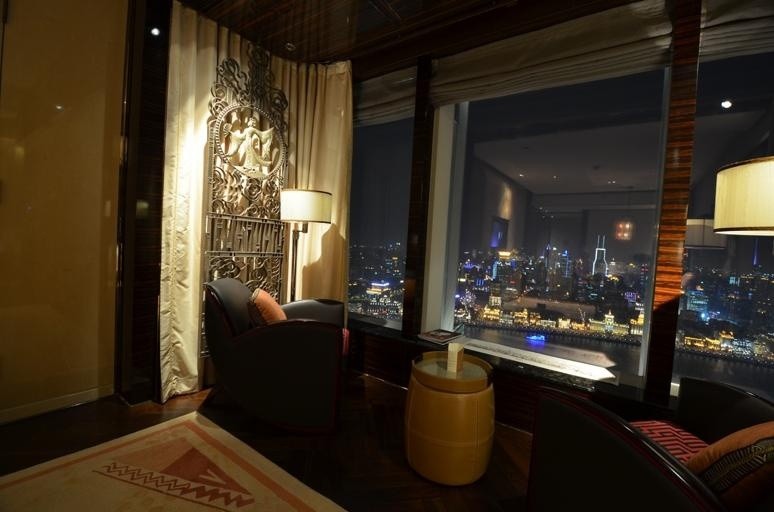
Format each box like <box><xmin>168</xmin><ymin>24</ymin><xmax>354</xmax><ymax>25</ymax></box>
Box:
<box><xmin>0</xmin><ymin>410</ymin><xmax>348</xmax><ymax>512</ymax></box>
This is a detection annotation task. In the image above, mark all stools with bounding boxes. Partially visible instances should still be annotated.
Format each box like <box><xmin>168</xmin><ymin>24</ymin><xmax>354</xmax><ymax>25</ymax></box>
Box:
<box><xmin>402</xmin><ymin>349</ymin><xmax>500</xmax><ymax>489</ymax></box>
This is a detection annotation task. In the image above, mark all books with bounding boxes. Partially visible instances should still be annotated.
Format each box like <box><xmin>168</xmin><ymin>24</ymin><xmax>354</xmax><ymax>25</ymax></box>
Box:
<box><xmin>417</xmin><ymin>329</ymin><xmax>463</xmax><ymax>345</ymax></box>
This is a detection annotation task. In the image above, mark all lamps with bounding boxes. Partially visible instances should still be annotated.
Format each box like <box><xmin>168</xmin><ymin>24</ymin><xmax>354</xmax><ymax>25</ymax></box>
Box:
<box><xmin>712</xmin><ymin>156</ymin><xmax>774</xmax><ymax>238</ymax></box>
<box><xmin>280</xmin><ymin>188</ymin><xmax>333</xmax><ymax>303</ymax></box>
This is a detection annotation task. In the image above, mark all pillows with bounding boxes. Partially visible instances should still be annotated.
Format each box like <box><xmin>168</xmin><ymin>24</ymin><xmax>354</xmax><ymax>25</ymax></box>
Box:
<box><xmin>685</xmin><ymin>420</ymin><xmax>774</xmax><ymax>501</ymax></box>
<box><xmin>248</xmin><ymin>287</ymin><xmax>287</xmax><ymax>325</ymax></box>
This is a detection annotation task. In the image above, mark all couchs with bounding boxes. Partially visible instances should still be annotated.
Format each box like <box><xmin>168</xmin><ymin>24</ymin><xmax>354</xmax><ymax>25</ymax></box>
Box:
<box><xmin>530</xmin><ymin>375</ymin><xmax>774</xmax><ymax>512</ymax></box>
<box><xmin>203</xmin><ymin>275</ymin><xmax>356</xmax><ymax>421</ymax></box>
